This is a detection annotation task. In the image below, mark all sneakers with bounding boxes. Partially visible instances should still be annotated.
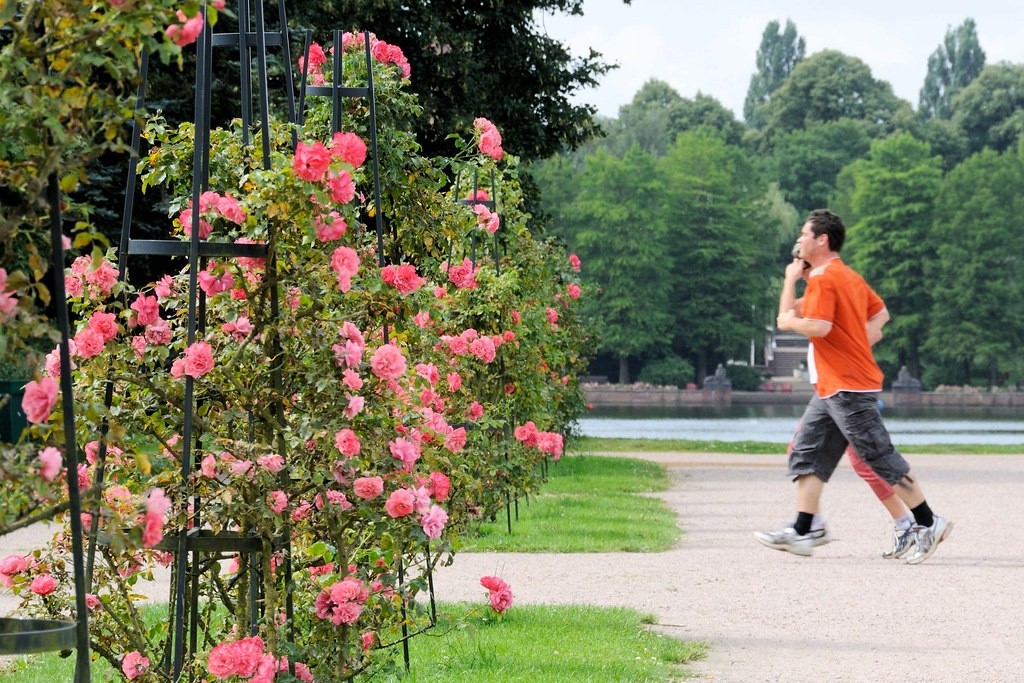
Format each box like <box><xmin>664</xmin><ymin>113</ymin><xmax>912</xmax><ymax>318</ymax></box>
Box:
<box><xmin>753</xmin><ymin>521</ymin><xmax>830</xmax><ymax>557</ymax></box>
<box><xmin>905</xmin><ymin>515</ymin><xmax>952</xmax><ymax>565</ymax></box>
<box><xmin>882</xmin><ymin>521</ymin><xmax>916</xmax><ymax>559</ymax></box>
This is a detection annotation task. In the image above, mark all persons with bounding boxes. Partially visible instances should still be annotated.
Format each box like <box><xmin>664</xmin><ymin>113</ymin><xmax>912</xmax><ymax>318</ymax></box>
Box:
<box><xmin>752</xmin><ymin>208</ymin><xmax>954</xmax><ymax>564</ymax></box>
